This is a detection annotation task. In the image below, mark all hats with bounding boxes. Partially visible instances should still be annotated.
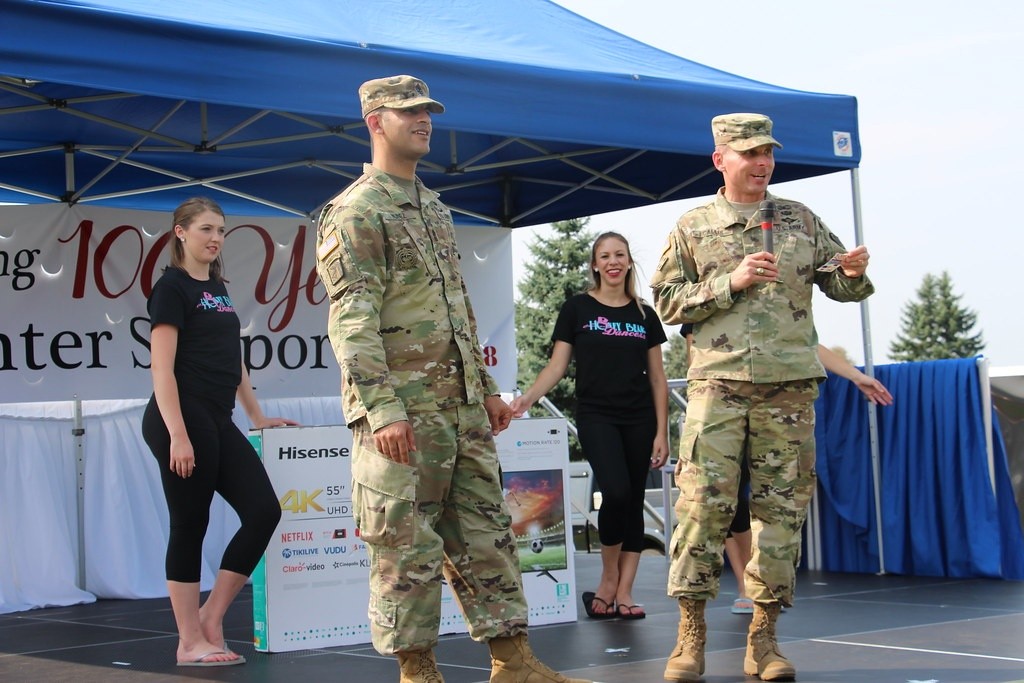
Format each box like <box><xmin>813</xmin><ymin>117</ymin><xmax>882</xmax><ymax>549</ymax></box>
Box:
<box><xmin>710</xmin><ymin>113</ymin><xmax>782</xmax><ymax>152</ymax></box>
<box><xmin>359</xmin><ymin>75</ymin><xmax>443</xmax><ymax>115</ymax></box>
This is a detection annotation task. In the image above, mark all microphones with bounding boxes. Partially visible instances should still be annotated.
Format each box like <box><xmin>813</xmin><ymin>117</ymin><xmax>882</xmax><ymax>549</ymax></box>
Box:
<box><xmin>758</xmin><ymin>199</ymin><xmax>775</xmax><ymax>264</ymax></box>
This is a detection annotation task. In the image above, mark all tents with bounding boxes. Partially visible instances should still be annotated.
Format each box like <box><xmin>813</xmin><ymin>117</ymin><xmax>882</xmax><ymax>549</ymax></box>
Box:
<box><xmin>0</xmin><ymin>0</ymin><xmax>889</xmax><ymax>601</ymax></box>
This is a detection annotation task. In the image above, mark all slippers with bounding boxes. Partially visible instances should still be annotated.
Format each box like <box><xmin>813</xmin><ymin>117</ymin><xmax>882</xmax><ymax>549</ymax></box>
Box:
<box><xmin>581</xmin><ymin>591</ymin><xmax>615</xmax><ymax>618</ymax></box>
<box><xmin>616</xmin><ymin>603</ymin><xmax>645</xmax><ymax>619</ymax></box>
<box><xmin>730</xmin><ymin>597</ymin><xmax>755</xmax><ymax>614</ymax></box>
<box><xmin>177</xmin><ymin>640</ymin><xmax>247</xmax><ymax>666</ymax></box>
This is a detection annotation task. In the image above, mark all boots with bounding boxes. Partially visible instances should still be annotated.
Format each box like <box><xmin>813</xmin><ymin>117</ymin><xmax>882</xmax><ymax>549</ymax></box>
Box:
<box><xmin>397</xmin><ymin>648</ymin><xmax>444</xmax><ymax>683</ymax></box>
<box><xmin>663</xmin><ymin>597</ymin><xmax>707</xmax><ymax>679</ymax></box>
<box><xmin>743</xmin><ymin>604</ymin><xmax>796</xmax><ymax>680</ymax></box>
<box><xmin>488</xmin><ymin>635</ymin><xmax>592</xmax><ymax>683</ymax></box>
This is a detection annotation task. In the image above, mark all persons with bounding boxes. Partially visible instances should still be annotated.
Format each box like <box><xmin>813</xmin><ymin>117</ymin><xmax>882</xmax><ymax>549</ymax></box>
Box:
<box><xmin>141</xmin><ymin>197</ymin><xmax>302</xmax><ymax>666</ymax></box>
<box><xmin>315</xmin><ymin>73</ymin><xmax>596</xmax><ymax>683</ymax></box>
<box><xmin>651</xmin><ymin>112</ymin><xmax>875</xmax><ymax>683</ymax></box>
<box><xmin>509</xmin><ymin>231</ymin><xmax>670</xmax><ymax>621</ymax></box>
<box><xmin>679</xmin><ymin>323</ymin><xmax>894</xmax><ymax>614</ymax></box>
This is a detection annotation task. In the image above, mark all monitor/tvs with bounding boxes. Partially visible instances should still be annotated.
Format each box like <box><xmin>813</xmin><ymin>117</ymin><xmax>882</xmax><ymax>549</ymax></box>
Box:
<box><xmin>501</xmin><ymin>469</ymin><xmax>567</xmax><ymax>582</ymax></box>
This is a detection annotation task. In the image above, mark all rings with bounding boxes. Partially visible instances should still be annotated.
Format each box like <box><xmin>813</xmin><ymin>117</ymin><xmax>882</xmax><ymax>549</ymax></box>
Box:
<box><xmin>861</xmin><ymin>260</ymin><xmax>865</xmax><ymax>267</ymax></box>
<box><xmin>756</xmin><ymin>267</ymin><xmax>765</xmax><ymax>277</ymax></box>
